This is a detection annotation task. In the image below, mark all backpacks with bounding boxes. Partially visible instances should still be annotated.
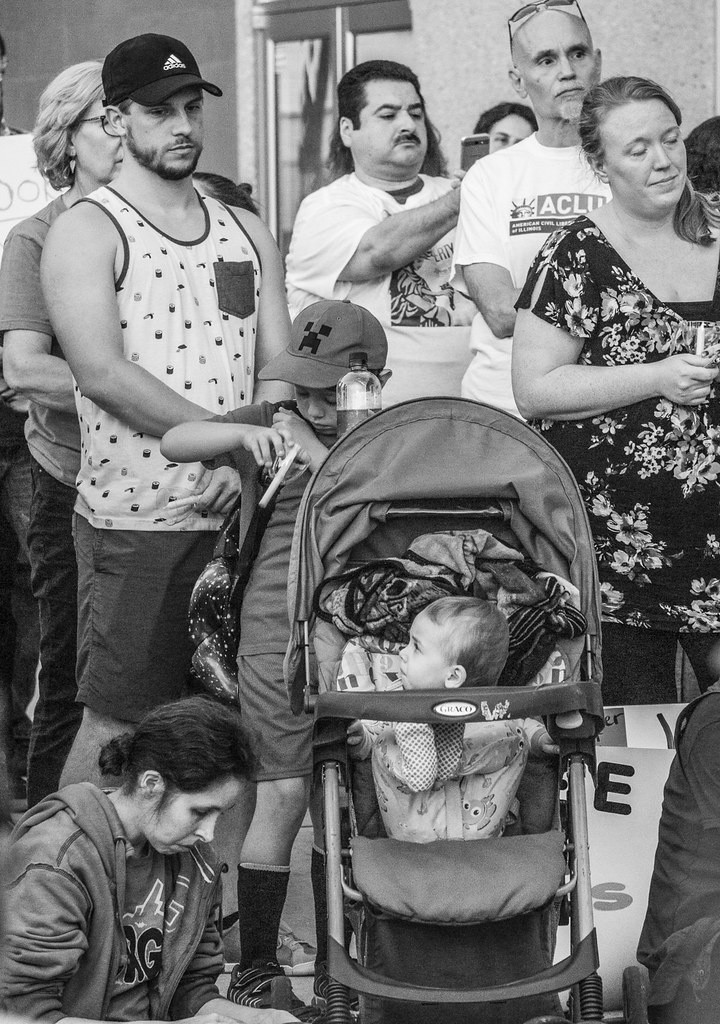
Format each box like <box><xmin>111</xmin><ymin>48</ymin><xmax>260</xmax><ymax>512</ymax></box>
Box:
<box><xmin>187</xmin><ymin>401</ymin><xmax>305</xmax><ymax>706</ymax></box>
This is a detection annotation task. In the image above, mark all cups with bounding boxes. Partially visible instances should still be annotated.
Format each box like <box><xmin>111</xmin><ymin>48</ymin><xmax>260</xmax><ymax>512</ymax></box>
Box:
<box><xmin>682</xmin><ymin>321</ymin><xmax>720</xmax><ymax>367</ymax></box>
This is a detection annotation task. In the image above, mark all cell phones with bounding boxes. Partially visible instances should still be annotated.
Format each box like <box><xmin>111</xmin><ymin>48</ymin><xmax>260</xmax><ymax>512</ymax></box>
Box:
<box><xmin>460</xmin><ymin>133</ymin><xmax>490</xmax><ymax>181</ymax></box>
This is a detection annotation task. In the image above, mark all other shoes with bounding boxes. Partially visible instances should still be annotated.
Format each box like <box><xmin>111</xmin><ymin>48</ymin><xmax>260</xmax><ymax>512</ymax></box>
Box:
<box><xmin>226</xmin><ymin>960</ymin><xmax>305</xmax><ymax>1016</ymax></box>
<box><xmin>310</xmin><ymin>960</ymin><xmax>361</xmax><ymax>1011</ymax></box>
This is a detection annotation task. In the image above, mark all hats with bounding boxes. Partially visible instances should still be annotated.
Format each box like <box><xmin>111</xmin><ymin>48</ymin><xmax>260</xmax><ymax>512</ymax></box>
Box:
<box><xmin>102</xmin><ymin>33</ymin><xmax>223</xmax><ymax>107</ymax></box>
<box><xmin>257</xmin><ymin>299</ymin><xmax>388</xmax><ymax>389</ymax></box>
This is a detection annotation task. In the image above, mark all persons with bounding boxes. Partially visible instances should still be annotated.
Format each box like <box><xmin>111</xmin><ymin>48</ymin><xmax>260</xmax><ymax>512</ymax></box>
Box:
<box><xmin>346</xmin><ymin>597</ymin><xmax>560</xmax><ymax>844</ymax></box>
<box><xmin>162</xmin><ymin>302</ymin><xmax>391</xmax><ymax>1024</ymax></box>
<box><xmin>285</xmin><ymin>0</ymin><xmax>720</xmax><ymax>705</ymax></box>
<box><xmin>0</xmin><ymin>31</ymin><xmax>293</xmax><ymax>819</ymax></box>
<box><xmin>0</xmin><ymin>696</ymin><xmax>298</xmax><ymax>1024</ymax></box>
<box><xmin>636</xmin><ymin>684</ymin><xmax>720</xmax><ymax>1024</ymax></box>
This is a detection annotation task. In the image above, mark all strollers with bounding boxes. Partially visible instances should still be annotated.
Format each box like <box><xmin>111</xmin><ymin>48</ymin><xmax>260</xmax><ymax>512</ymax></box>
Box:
<box><xmin>271</xmin><ymin>397</ymin><xmax>650</xmax><ymax>1024</ymax></box>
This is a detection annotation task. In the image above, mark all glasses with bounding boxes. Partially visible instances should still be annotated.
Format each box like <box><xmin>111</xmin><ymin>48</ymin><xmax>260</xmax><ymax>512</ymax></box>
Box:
<box><xmin>508</xmin><ymin>0</ymin><xmax>586</xmax><ymax>53</ymax></box>
<box><xmin>78</xmin><ymin>114</ymin><xmax>122</xmax><ymax>137</ymax></box>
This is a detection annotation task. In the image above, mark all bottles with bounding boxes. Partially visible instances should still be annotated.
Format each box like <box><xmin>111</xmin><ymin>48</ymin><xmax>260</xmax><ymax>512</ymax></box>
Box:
<box><xmin>336</xmin><ymin>353</ymin><xmax>383</xmax><ymax>442</ymax></box>
